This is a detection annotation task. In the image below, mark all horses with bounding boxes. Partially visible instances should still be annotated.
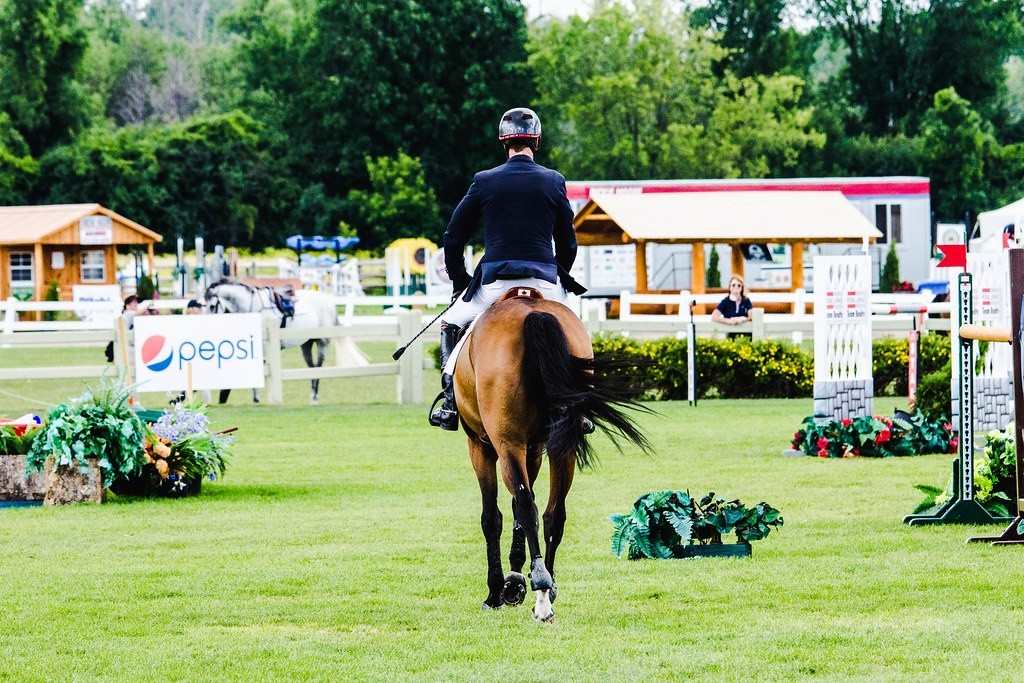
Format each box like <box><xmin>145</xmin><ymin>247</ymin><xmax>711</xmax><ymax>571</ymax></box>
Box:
<box><xmin>203</xmin><ymin>277</ymin><xmax>376</xmax><ymax>407</ymax></box>
<box><xmin>429</xmin><ymin>283</ymin><xmax>670</xmax><ymax>625</ymax></box>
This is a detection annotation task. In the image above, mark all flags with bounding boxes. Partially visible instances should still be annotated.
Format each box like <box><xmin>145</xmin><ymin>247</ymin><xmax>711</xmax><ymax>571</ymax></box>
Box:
<box><xmin>936</xmin><ymin>245</ymin><xmax>966</xmax><ymax>267</ymax></box>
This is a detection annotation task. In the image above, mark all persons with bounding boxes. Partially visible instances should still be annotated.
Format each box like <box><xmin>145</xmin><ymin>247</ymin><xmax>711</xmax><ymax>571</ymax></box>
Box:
<box><xmin>710</xmin><ymin>275</ymin><xmax>754</xmax><ymax>341</ymax></box>
<box><xmin>121</xmin><ymin>295</ymin><xmax>153</xmax><ymax>384</ymax></box>
<box><xmin>427</xmin><ymin>108</ymin><xmax>595</xmax><ymax>434</ymax></box>
<box><xmin>168</xmin><ymin>299</ymin><xmax>206</xmax><ymax>407</ymax></box>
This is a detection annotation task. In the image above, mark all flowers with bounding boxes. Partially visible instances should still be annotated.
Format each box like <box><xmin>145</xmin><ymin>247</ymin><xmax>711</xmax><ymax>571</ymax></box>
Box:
<box><xmin>790</xmin><ymin>412</ymin><xmax>960</xmax><ymax>460</ymax></box>
<box><xmin>0</xmin><ymin>361</ymin><xmax>240</xmax><ymax>494</ymax></box>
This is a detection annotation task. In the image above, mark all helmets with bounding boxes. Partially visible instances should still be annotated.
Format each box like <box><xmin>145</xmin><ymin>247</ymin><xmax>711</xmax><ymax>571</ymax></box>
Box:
<box><xmin>499</xmin><ymin>107</ymin><xmax>542</xmax><ymax>141</ymax></box>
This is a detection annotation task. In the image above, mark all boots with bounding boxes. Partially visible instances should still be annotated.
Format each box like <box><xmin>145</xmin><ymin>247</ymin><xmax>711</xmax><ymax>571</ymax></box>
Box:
<box><xmin>431</xmin><ymin>323</ymin><xmax>460</xmax><ymax>432</ymax></box>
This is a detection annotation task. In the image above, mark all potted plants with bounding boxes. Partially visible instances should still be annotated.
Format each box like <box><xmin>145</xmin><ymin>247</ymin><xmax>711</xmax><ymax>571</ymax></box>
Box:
<box><xmin>608</xmin><ymin>491</ymin><xmax>784</xmax><ymax>560</ymax></box>
<box><xmin>914</xmin><ymin>421</ymin><xmax>1017</xmax><ymax>516</ymax></box>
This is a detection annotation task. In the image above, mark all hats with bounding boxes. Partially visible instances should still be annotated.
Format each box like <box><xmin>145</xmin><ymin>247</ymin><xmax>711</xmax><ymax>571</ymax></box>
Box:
<box><xmin>188</xmin><ymin>300</ymin><xmax>206</xmax><ymax>308</ymax></box>
<box><xmin>123</xmin><ymin>293</ymin><xmax>143</xmax><ymax>306</ymax></box>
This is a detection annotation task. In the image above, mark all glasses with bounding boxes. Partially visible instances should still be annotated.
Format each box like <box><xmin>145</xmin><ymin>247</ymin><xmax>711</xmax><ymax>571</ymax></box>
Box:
<box><xmin>731</xmin><ymin>284</ymin><xmax>742</xmax><ymax>287</ymax></box>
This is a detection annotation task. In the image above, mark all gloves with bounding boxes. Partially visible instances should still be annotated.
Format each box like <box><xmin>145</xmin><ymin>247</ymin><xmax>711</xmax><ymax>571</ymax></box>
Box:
<box><xmin>452</xmin><ymin>275</ymin><xmax>473</xmax><ymax>294</ymax></box>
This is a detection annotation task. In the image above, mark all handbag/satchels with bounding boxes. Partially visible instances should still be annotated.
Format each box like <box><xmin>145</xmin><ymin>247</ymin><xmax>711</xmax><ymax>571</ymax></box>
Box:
<box><xmin>105</xmin><ymin>341</ymin><xmax>115</xmax><ymax>362</ymax></box>
